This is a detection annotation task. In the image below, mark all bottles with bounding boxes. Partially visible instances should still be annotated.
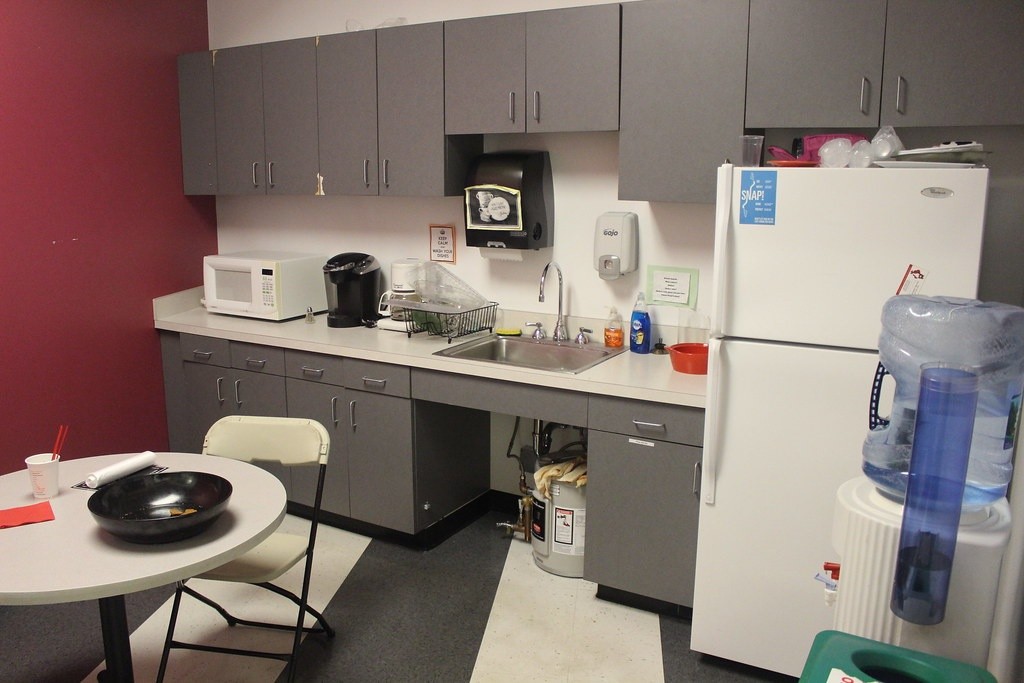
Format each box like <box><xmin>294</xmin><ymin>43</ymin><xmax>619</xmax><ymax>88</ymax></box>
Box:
<box><xmin>305</xmin><ymin>306</ymin><xmax>315</xmax><ymax>323</ymax></box>
<box><xmin>861</xmin><ymin>292</ymin><xmax>1024</xmax><ymax>504</ymax></box>
<box><xmin>603</xmin><ymin>306</ymin><xmax>623</xmax><ymax>348</ymax></box>
<box><xmin>630</xmin><ymin>291</ymin><xmax>651</xmax><ymax>354</ymax></box>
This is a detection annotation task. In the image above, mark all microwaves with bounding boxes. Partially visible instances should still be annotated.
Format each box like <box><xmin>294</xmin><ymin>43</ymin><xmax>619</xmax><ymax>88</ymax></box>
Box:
<box><xmin>203</xmin><ymin>250</ymin><xmax>328</xmax><ymax>320</ymax></box>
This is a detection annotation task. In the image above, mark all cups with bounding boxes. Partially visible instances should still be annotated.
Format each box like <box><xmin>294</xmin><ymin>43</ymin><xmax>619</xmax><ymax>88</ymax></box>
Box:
<box><xmin>24</xmin><ymin>453</ymin><xmax>61</xmax><ymax>499</ymax></box>
<box><xmin>738</xmin><ymin>135</ymin><xmax>764</xmax><ymax>167</ymax></box>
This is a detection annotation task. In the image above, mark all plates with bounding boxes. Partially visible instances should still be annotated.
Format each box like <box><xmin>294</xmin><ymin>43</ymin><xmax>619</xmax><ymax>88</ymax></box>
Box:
<box><xmin>890</xmin><ymin>151</ymin><xmax>992</xmax><ymax>164</ymax></box>
<box><xmin>767</xmin><ymin>160</ymin><xmax>818</xmax><ymax>166</ymax></box>
<box><xmin>873</xmin><ymin>160</ymin><xmax>975</xmax><ymax>168</ymax></box>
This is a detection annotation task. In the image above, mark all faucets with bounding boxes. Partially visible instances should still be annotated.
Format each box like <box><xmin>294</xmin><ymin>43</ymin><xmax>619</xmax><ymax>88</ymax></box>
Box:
<box><xmin>538</xmin><ymin>260</ymin><xmax>568</xmax><ymax>342</ymax></box>
<box><xmin>823</xmin><ymin>561</ymin><xmax>841</xmax><ymax>583</ymax></box>
<box><xmin>813</xmin><ymin>572</ymin><xmax>838</xmax><ymax>607</ymax></box>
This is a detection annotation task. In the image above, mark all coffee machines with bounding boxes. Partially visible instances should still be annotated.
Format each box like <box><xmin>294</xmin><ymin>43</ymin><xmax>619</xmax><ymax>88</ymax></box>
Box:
<box><xmin>322</xmin><ymin>251</ymin><xmax>381</xmax><ymax>328</ymax></box>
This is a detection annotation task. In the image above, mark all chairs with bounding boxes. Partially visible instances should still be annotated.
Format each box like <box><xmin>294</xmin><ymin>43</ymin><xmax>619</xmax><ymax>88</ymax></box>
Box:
<box><xmin>156</xmin><ymin>414</ymin><xmax>335</xmax><ymax>683</ymax></box>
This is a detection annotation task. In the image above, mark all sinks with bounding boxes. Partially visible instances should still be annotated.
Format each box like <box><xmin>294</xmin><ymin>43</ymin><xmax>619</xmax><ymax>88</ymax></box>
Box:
<box><xmin>432</xmin><ymin>332</ymin><xmax>631</xmax><ymax>375</ymax></box>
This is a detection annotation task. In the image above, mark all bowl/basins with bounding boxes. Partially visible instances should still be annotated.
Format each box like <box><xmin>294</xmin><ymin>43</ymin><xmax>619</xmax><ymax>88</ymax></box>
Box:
<box><xmin>87</xmin><ymin>471</ymin><xmax>234</xmax><ymax>544</ymax></box>
<box><xmin>665</xmin><ymin>343</ymin><xmax>708</xmax><ymax>375</ymax></box>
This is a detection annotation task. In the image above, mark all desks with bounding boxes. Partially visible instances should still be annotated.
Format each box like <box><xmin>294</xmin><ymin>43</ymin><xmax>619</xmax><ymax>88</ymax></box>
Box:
<box><xmin>0</xmin><ymin>452</ymin><xmax>286</xmax><ymax>683</ymax></box>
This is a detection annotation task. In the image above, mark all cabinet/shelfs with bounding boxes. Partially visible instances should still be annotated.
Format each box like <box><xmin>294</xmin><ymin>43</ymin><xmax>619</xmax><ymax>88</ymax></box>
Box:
<box><xmin>583</xmin><ymin>393</ymin><xmax>706</xmax><ymax>620</ymax></box>
<box><xmin>179</xmin><ymin>332</ymin><xmax>493</xmax><ymax>552</ymax></box>
<box><xmin>177</xmin><ymin>0</ymin><xmax>1024</xmax><ymax>202</ymax></box>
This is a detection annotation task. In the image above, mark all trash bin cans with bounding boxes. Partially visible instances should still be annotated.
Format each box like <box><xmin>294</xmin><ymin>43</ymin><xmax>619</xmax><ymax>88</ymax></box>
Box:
<box><xmin>796</xmin><ymin>630</ymin><xmax>998</xmax><ymax>683</ymax></box>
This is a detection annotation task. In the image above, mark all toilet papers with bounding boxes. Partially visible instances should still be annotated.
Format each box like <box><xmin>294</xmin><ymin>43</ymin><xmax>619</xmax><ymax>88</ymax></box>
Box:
<box><xmin>86</xmin><ymin>450</ymin><xmax>159</xmax><ymax>488</ymax></box>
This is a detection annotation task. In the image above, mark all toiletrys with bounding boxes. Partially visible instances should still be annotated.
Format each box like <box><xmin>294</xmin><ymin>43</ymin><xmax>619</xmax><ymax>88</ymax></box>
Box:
<box><xmin>604</xmin><ymin>305</ymin><xmax>624</xmax><ymax>348</ymax></box>
<box><xmin>629</xmin><ymin>291</ymin><xmax>651</xmax><ymax>354</ymax></box>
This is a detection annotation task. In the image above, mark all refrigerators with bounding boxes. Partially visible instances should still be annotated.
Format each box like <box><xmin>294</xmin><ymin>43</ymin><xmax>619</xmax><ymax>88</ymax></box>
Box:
<box><xmin>687</xmin><ymin>160</ymin><xmax>988</xmax><ymax>679</ymax></box>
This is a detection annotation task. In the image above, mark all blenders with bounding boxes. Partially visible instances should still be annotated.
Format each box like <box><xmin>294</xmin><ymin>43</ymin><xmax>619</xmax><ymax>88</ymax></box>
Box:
<box><xmin>377</xmin><ymin>259</ymin><xmax>425</xmax><ymax>334</ymax></box>
